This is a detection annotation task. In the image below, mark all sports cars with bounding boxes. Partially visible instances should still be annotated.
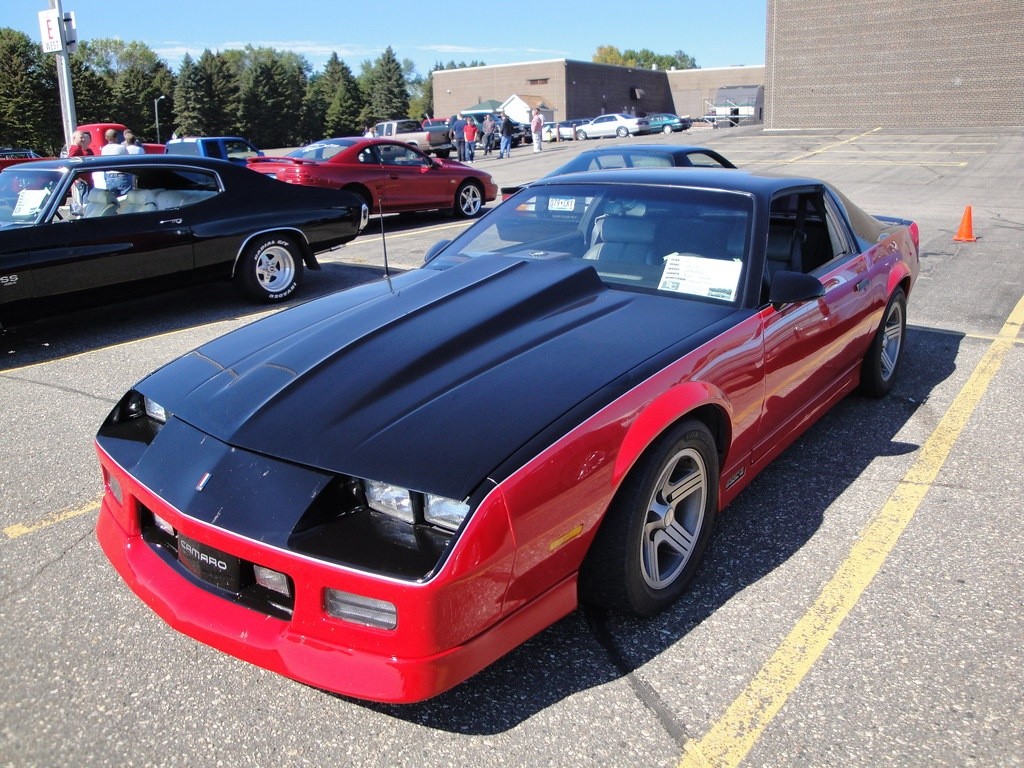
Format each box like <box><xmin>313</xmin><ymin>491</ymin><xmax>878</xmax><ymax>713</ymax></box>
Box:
<box><xmin>245</xmin><ymin>138</ymin><xmax>499</xmax><ymax>233</ymax></box>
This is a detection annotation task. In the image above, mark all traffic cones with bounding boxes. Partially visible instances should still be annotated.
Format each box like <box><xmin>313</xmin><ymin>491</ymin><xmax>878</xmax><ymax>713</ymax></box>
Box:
<box><xmin>952</xmin><ymin>205</ymin><xmax>977</xmax><ymax>242</ymax></box>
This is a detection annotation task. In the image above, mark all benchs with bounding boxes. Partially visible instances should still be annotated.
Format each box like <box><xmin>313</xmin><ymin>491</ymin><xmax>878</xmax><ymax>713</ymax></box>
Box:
<box><xmin>666</xmin><ymin>219</ymin><xmax>834</xmax><ymax>276</ymax></box>
<box><xmin>145</xmin><ymin>187</ymin><xmax>217</xmax><ymax>210</ymax></box>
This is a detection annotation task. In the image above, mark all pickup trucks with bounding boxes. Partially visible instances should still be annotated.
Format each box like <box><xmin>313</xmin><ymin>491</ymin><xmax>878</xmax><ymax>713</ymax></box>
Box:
<box><xmin>362</xmin><ymin>119</ymin><xmax>457</xmax><ymax>158</ymax></box>
<box><xmin>0</xmin><ymin>122</ymin><xmax>166</xmax><ymax>172</ymax></box>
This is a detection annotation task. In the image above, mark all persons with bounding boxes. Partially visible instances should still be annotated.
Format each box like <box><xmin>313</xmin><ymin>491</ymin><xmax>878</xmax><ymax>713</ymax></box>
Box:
<box><xmin>68</xmin><ymin>132</ymin><xmax>95</xmax><ymax>204</ymax></box>
<box><xmin>453</xmin><ymin>113</ymin><xmax>478</xmax><ymax>163</ymax></box>
<box><xmin>363</xmin><ymin>126</ymin><xmax>378</xmax><ymax>153</ymax></box>
<box><xmin>497</xmin><ymin>114</ymin><xmax>513</xmax><ymax>159</ymax></box>
<box><xmin>101</xmin><ymin>129</ymin><xmax>144</xmax><ymax>194</ymax></box>
<box><xmin>531</xmin><ymin>108</ymin><xmax>543</xmax><ymax>152</ymax></box>
<box><xmin>483</xmin><ymin>115</ymin><xmax>496</xmax><ymax>155</ymax></box>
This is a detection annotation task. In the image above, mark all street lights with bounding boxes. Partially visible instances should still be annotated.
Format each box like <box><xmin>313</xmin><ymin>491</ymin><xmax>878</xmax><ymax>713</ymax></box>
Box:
<box><xmin>154</xmin><ymin>95</ymin><xmax>165</xmax><ymax>144</ymax></box>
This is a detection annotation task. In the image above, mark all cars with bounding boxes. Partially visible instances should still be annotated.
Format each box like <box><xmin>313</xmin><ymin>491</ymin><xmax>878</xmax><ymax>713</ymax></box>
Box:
<box><xmin>0</xmin><ymin>147</ymin><xmax>42</xmax><ymax>159</ymax></box>
<box><xmin>92</xmin><ymin>167</ymin><xmax>921</xmax><ymax>691</ymax></box>
<box><xmin>570</xmin><ymin>113</ymin><xmax>651</xmax><ymax>141</ymax></box>
<box><xmin>632</xmin><ymin>113</ymin><xmax>692</xmax><ymax>137</ymax></box>
<box><xmin>448</xmin><ymin>112</ymin><xmax>600</xmax><ymax>151</ymax></box>
<box><xmin>496</xmin><ymin>143</ymin><xmax>738</xmax><ymax>243</ymax></box>
<box><xmin>0</xmin><ymin>154</ymin><xmax>371</xmax><ymax>329</ymax></box>
<box><xmin>422</xmin><ymin>118</ymin><xmax>451</xmax><ymax>127</ymax></box>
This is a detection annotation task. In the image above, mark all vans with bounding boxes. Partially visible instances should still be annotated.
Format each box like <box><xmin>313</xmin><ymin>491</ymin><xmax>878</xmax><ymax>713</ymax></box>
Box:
<box><xmin>164</xmin><ymin>136</ymin><xmax>266</xmax><ymax>168</ymax></box>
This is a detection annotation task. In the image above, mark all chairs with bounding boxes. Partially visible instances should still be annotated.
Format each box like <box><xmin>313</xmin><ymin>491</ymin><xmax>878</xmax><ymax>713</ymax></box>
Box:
<box><xmin>364</xmin><ymin>152</ymin><xmax>396</xmax><ymax>163</ymax></box>
<box><xmin>581</xmin><ymin>216</ymin><xmax>671</xmax><ymax>265</ymax></box>
<box><xmin>116</xmin><ymin>189</ymin><xmax>157</xmax><ymax>215</ymax></box>
<box><xmin>726</xmin><ymin>227</ymin><xmax>802</xmax><ymax>284</ymax></box>
<box><xmin>82</xmin><ymin>188</ymin><xmax>116</xmax><ymax>218</ymax></box>
